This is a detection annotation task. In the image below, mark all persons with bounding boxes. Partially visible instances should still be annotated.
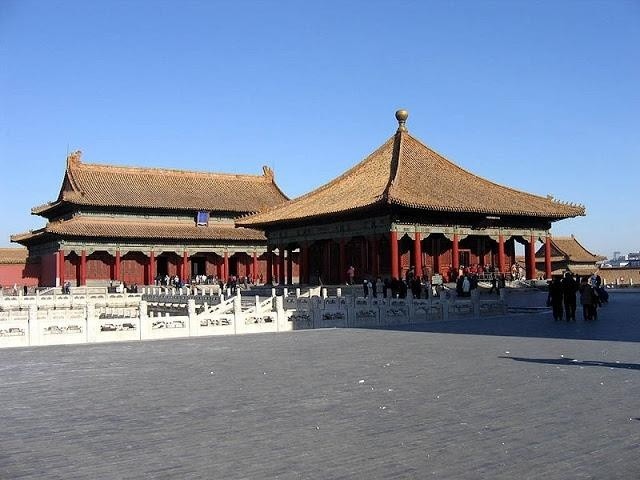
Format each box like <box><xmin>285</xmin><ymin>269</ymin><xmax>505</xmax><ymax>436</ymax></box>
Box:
<box><xmin>24</xmin><ymin>283</ymin><xmax>28</xmax><ymax>295</ymax></box>
<box><xmin>156</xmin><ymin>272</ymin><xmax>263</xmax><ymax>294</ymax></box>
<box><xmin>363</xmin><ymin>262</ymin><xmax>522</xmax><ymax>299</ymax></box>
<box><xmin>347</xmin><ymin>266</ymin><xmax>356</xmax><ymax>285</ymax></box>
<box><xmin>66</xmin><ymin>282</ymin><xmax>71</xmax><ymax>295</ymax></box>
<box><xmin>120</xmin><ymin>279</ymin><xmax>137</xmax><ymax>293</ymax></box>
<box><xmin>548</xmin><ymin>271</ymin><xmax>601</xmax><ymax>321</ymax></box>
<box><xmin>13</xmin><ymin>283</ymin><xmax>18</xmax><ymax>296</ymax></box>
<box><xmin>63</xmin><ymin>282</ymin><xmax>67</xmax><ymax>294</ymax></box>
